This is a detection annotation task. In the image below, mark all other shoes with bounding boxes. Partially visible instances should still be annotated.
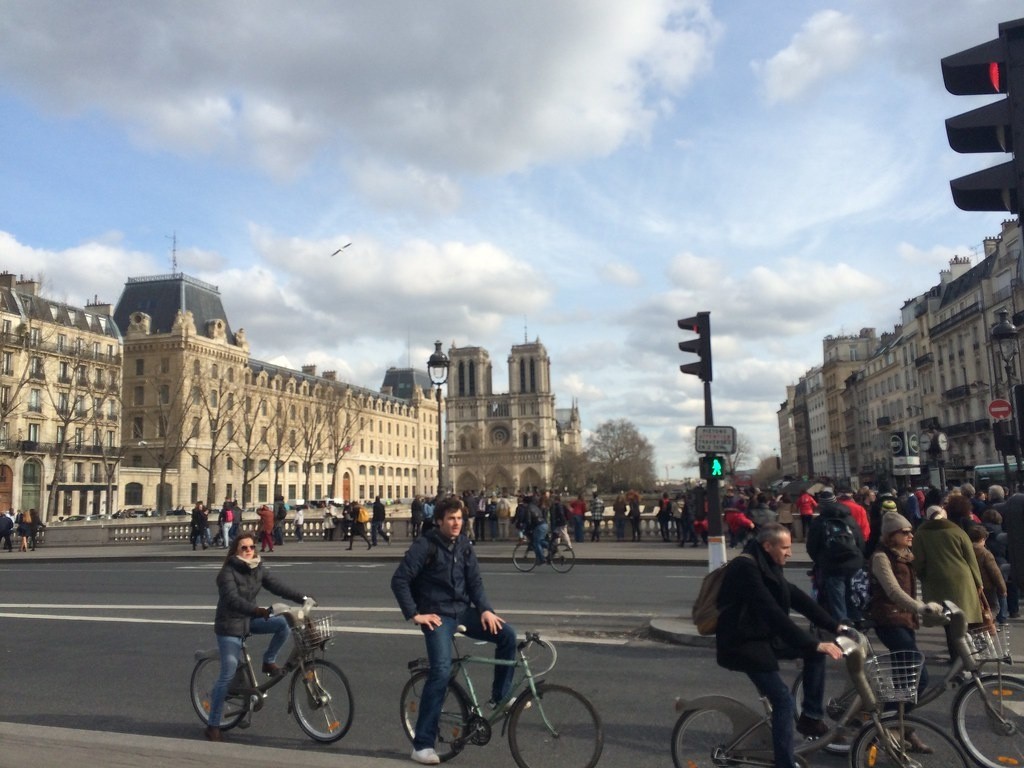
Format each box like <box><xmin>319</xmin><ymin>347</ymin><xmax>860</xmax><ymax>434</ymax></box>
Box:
<box><xmin>907</xmin><ymin>730</ymin><xmax>935</xmax><ymax>754</ymax></box>
<box><xmin>205</xmin><ymin>726</ymin><xmax>221</xmax><ymax>742</ymax></box>
<box><xmin>260</xmin><ymin>549</ymin><xmax>274</xmax><ymax>553</ymax></box>
<box><xmin>262</xmin><ymin>663</ymin><xmax>279</xmax><ymax>673</ymax></box>
<box><xmin>795</xmin><ymin>717</ymin><xmax>846</xmax><ymax>744</ymax></box>
<box><xmin>537</xmin><ymin>556</ymin><xmax>547</xmax><ymax>566</ymax></box>
<box><xmin>563</xmin><ymin>547</ymin><xmax>572</xmax><ymax>552</ymax></box>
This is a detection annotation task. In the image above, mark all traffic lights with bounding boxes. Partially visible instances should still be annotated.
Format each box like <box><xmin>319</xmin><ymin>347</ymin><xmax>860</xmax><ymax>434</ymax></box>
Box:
<box><xmin>698</xmin><ymin>456</ymin><xmax>726</xmax><ymax>480</ymax></box>
<box><xmin>940</xmin><ymin>15</ymin><xmax>1023</xmax><ymax>222</ymax></box>
<box><xmin>677</xmin><ymin>310</ymin><xmax>713</xmax><ymax>383</ymax></box>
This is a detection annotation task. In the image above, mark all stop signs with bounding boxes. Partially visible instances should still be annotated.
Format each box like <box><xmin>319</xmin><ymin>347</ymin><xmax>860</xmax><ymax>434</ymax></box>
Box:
<box><xmin>988</xmin><ymin>399</ymin><xmax>1012</xmax><ymax>420</ymax></box>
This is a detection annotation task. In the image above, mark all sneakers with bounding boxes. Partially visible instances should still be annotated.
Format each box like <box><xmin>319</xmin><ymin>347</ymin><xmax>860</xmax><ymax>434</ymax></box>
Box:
<box><xmin>488</xmin><ymin>698</ymin><xmax>533</xmax><ymax>712</ymax></box>
<box><xmin>409</xmin><ymin>748</ymin><xmax>440</xmax><ymax>764</ymax></box>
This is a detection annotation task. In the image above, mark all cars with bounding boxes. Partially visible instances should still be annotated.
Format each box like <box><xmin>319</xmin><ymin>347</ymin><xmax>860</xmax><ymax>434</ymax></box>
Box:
<box><xmin>972</xmin><ymin>462</ymin><xmax>1024</xmax><ymax>500</ymax></box>
<box><xmin>58</xmin><ymin>498</ymin><xmax>348</xmax><ymax>521</ymax></box>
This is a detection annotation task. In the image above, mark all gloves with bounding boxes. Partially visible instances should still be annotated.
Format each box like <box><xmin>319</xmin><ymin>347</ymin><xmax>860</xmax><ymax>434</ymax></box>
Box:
<box><xmin>920</xmin><ymin>602</ymin><xmax>944</xmax><ymax>616</ymax></box>
<box><xmin>252</xmin><ymin>608</ymin><xmax>270</xmax><ymax>621</ymax></box>
<box><xmin>303</xmin><ymin>594</ymin><xmax>318</xmax><ymax>607</ymax></box>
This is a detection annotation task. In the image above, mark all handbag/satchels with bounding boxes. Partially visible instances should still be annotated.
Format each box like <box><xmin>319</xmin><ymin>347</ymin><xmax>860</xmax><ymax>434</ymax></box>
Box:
<box><xmin>968</xmin><ymin>589</ymin><xmax>997</xmax><ymax>636</ymax></box>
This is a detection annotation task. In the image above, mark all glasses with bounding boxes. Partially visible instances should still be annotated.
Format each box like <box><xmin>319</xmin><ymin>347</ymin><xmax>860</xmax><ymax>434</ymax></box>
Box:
<box><xmin>241</xmin><ymin>545</ymin><xmax>256</xmax><ymax>551</ymax></box>
<box><xmin>897</xmin><ymin>530</ymin><xmax>913</xmax><ymax>536</ymax></box>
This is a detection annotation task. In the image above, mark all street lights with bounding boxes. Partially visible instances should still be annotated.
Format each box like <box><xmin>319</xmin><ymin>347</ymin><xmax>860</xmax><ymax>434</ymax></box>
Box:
<box><xmin>427</xmin><ymin>339</ymin><xmax>450</xmax><ymax>494</ymax></box>
<box><xmin>991</xmin><ymin>307</ymin><xmax>1024</xmax><ymax>493</ymax></box>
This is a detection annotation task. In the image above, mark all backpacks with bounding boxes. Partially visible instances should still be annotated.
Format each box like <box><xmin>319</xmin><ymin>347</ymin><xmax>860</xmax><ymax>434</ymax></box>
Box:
<box><xmin>356</xmin><ymin>507</ymin><xmax>369</xmax><ymax>523</ymax></box>
<box><xmin>224</xmin><ymin>509</ymin><xmax>234</xmax><ymax>522</ymax></box>
<box><xmin>848</xmin><ymin>550</ymin><xmax>895</xmax><ymax>618</ymax></box>
<box><xmin>477</xmin><ymin>496</ymin><xmax>486</xmax><ymax>512</ymax></box>
<box><xmin>692</xmin><ymin>554</ymin><xmax>757</xmax><ymax>635</ymax></box>
<box><xmin>820</xmin><ymin>515</ymin><xmax>865</xmax><ymax>572</ymax></box>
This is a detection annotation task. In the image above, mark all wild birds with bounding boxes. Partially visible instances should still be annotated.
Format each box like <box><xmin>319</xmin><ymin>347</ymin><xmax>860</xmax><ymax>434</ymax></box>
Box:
<box><xmin>332</xmin><ymin>243</ymin><xmax>350</xmax><ymax>256</ymax></box>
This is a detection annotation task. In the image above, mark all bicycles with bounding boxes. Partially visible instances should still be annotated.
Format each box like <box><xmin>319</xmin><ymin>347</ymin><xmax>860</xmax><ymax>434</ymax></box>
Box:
<box><xmin>670</xmin><ymin>621</ymin><xmax>968</xmax><ymax>768</ymax></box>
<box><xmin>791</xmin><ymin>597</ymin><xmax>1024</xmax><ymax>767</ymax></box>
<box><xmin>511</xmin><ymin>537</ymin><xmax>576</xmax><ymax>573</ymax></box>
<box><xmin>188</xmin><ymin>605</ymin><xmax>358</xmax><ymax>742</ymax></box>
<box><xmin>400</xmin><ymin>631</ymin><xmax>607</xmax><ymax>768</ymax></box>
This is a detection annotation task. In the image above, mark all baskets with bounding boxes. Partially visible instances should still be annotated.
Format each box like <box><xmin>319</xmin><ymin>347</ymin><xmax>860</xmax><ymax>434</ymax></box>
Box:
<box><xmin>969</xmin><ymin>624</ymin><xmax>1010</xmax><ymax>660</ymax></box>
<box><xmin>871</xmin><ymin>650</ymin><xmax>926</xmax><ymax>702</ymax></box>
<box><xmin>293</xmin><ymin>613</ymin><xmax>335</xmax><ymax>649</ymax></box>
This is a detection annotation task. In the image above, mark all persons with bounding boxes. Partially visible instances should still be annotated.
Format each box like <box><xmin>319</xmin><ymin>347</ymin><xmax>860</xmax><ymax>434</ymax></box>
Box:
<box><xmin>657</xmin><ymin>478</ymin><xmax>1024</xmax><ymax>767</ymax></box>
<box><xmin>0</xmin><ymin>507</ymin><xmax>46</xmax><ymax>552</ymax></box>
<box><xmin>115</xmin><ymin>496</ymin><xmax>393</xmax><ymax>552</ymax></box>
<box><xmin>205</xmin><ymin>534</ymin><xmax>312</xmax><ymax>743</ymax></box>
<box><xmin>390</xmin><ymin>497</ymin><xmax>533</xmax><ymax>765</ymax></box>
<box><xmin>411</xmin><ymin>485</ymin><xmax>641</xmax><ymax>566</ymax></box>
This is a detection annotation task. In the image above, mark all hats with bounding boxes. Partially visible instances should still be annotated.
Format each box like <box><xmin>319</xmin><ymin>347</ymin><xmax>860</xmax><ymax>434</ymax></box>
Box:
<box><xmin>819</xmin><ymin>492</ymin><xmax>837</xmax><ymax>509</ymax></box>
<box><xmin>926</xmin><ymin>506</ymin><xmax>947</xmax><ymax>519</ymax></box>
<box><xmin>880</xmin><ymin>500</ymin><xmax>912</xmax><ymax>537</ymax></box>
<box><xmin>982</xmin><ymin>509</ymin><xmax>1002</xmax><ymax>524</ymax></box>
